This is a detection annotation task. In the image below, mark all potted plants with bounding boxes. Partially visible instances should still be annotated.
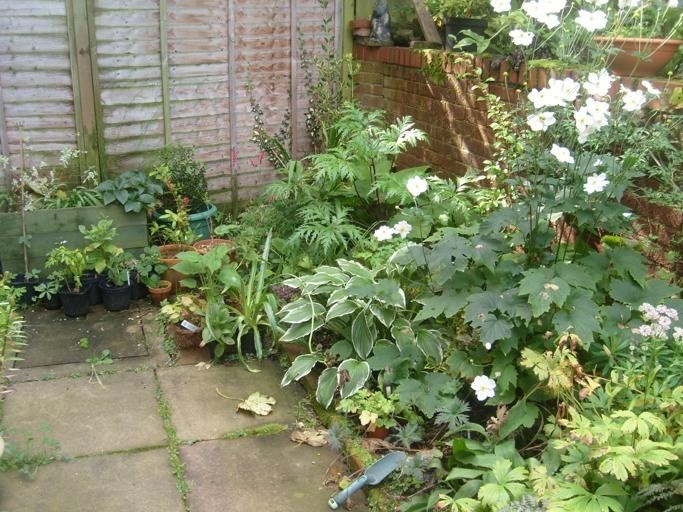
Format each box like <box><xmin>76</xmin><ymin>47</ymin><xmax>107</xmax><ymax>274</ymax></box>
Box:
<box><xmin>386</xmin><ymin>0</ymin><xmax>682</xmax><ymax>79</ymax></box>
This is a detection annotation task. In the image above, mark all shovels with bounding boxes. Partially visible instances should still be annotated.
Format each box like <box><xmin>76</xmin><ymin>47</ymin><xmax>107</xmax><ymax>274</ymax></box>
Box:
<box><xmin>327</xmin><ymin>450</ymin><xmax>406</xmax><ymax>510</ymax></box>
